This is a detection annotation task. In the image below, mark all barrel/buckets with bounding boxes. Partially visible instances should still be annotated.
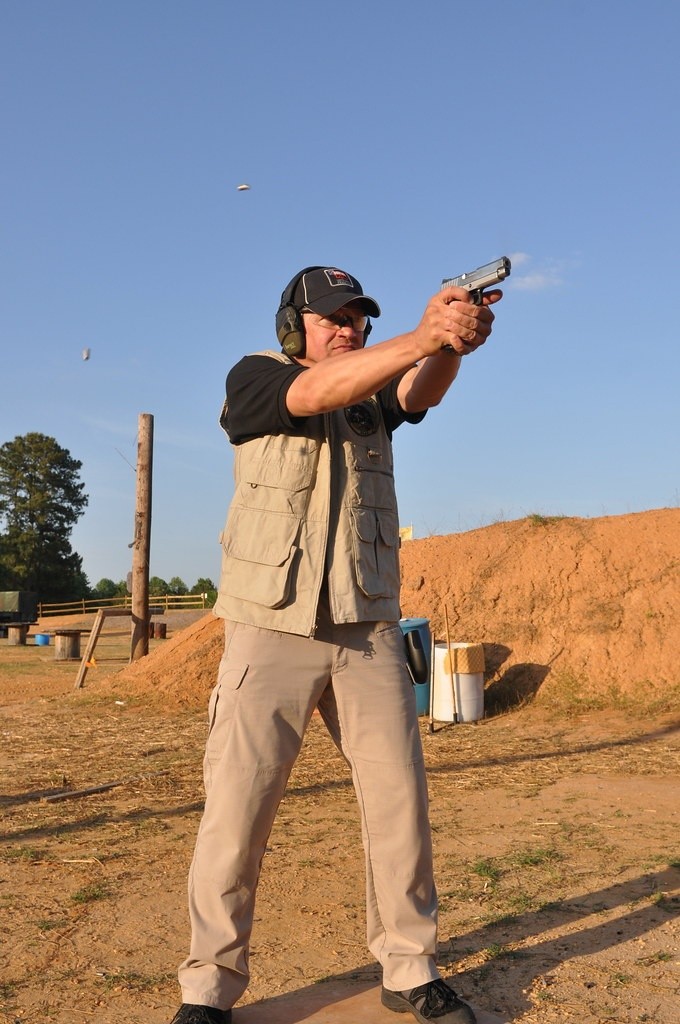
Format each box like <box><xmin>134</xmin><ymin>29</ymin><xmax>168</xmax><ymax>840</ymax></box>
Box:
<box><xmin>401</xmin><ymin>616</ymin><xmax>484</xmax><ymax>723</ymax></box>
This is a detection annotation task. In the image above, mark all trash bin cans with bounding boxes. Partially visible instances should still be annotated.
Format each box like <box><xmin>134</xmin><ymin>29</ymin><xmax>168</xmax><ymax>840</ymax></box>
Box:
<box><xmin>397</xmin><ymin>617</ymin><xmax>431</xmax><ymax>717</ymax></box>
<box><xmin>429</xmin><ymin>642</ymin><xmax>484</xmax><ymax>722</ymax></box>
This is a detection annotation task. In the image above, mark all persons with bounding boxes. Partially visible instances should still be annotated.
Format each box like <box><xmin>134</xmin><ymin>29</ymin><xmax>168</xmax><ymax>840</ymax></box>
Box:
<box><xmin>169</xmin><ymin>267</ymin><xmax>502</xmax><ymax>1023</ymax></box>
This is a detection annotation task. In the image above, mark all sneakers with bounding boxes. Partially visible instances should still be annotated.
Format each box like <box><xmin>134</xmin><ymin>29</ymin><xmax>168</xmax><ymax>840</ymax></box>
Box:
<box><xmin>170</xmin><ymin>1003</ymin><xmax>232</xmax><ymax>1024</ymax></box>
<box><xmin>381</xmin><ymin>978</ymin><xmax>477</xmax><ymax>1024</ymax></box>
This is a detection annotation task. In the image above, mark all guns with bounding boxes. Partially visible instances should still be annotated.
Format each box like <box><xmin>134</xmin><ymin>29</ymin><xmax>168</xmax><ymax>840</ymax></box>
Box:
<box><xmin>439</xmin><ymin>255</ymin><xmax>512</xmax><ymax>356</ymax></box>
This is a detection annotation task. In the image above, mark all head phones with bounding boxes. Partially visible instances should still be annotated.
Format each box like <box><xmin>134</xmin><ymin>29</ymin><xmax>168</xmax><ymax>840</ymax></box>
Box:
<box><xmin>275</xmin><ymin>266</ymin><xmax>372</xmax><ymax>356</ymax></box>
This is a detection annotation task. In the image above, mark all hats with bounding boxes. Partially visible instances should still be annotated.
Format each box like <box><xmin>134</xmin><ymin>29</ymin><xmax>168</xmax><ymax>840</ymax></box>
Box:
<box><xmin>290</xmin><ymin>267</ymin><xmax>381</xmax><ymax>318</ymax></box>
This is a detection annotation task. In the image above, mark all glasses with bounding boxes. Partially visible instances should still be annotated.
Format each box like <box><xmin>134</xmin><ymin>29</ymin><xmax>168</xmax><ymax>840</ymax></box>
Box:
<box><xmin>301</xmin><ymin>306</ymin><xmax>369</xmax><ymax>332</ymax></box>
